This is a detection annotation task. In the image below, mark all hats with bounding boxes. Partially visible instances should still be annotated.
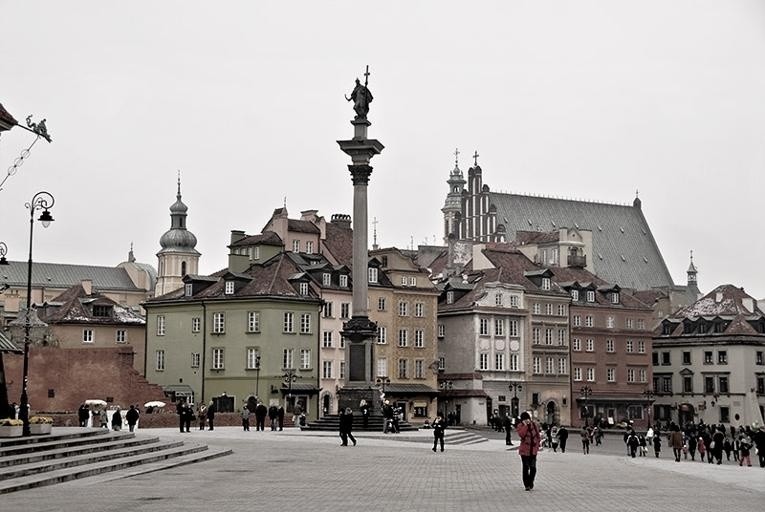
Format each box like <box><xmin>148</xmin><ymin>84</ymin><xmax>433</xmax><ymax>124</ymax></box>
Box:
<box><xmin>521</xmin><ymin>412</ymin><xmax>531</xmax><ymax>420</ymax></box>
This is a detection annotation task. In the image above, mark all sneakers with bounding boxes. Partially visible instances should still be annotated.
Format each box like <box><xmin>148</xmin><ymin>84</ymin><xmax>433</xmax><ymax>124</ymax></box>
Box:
<box><xmin>548</xmin><ymin>442</ymin><xmax>765</xmax><ymax>467</ymax></box>
<box><xmin>507</xmin><ymin>442</ymin><xmax>514</xmax><ymax>446</ymax></box>
<box><xmin>341</xmin><ymin>431</ymin><xmax>445</xmax><ymax>452</ymax></box>
<box><xmin>79</xmin><ymin>424</ymin><xmax>284</xmax><ymax>432</ymax></box>
<box><xmin>525</xmin><ymin>483</ymin><xmax>534</xmax><ymax>491</ymax></box>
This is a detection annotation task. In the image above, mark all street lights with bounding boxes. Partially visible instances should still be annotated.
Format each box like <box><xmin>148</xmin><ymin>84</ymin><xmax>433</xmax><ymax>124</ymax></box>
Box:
<box><xmin>0</xmin><ymin>241</ymin><xmax>10</xmax><ymax>266</ymax></box>
<box><xmin>16</xmin><ymin>190</ymin><xmax>56</xmax><ymax>438</ymax></box>
<box><xmin>506</xmin><ymin>382</ymin><xmax>522</xmax><ymax>418</ymax></box>
<box><xmin>438</xmin><ymin>380</ymin><xmax>453</xmax><ymax>423</ymax></box>
<box><xmin>282</xmin><ymin>371</ymin><xmax>298</xmax><ymax>411</ymax></box>
<box><xmin>642</xmin><ymin>390</ymin><xmax>656</xmax><ymax>430</ymax></box>
<box><xmin>374</xmin><ymin>376</ymin><xmax>391</xmax><ymax>394</ymax></box>
<box><xmin>580</xmin><ymin>385</ymin><xmax>593</xmax><ymax>429</ymax></box>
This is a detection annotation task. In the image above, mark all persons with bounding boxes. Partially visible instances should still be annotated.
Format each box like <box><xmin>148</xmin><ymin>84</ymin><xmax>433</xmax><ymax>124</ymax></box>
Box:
<box><xmin>490</xmin><ymin>411</ymin><xmax>568</xmax><ymax>453</ymax></box>
<box><xmin>448</xmin><ymin>409</ymin><xmax>460</xmax><ymax>426</ymax></box>
<box><xmin>360</xmin><ymin>400</ymin><xmax>370</xmax><ymax>428</ymax></box>
<box><xmin>9</xmin><ymin>402</ymin><xmax>31</xmax><ymax>419</ymax></box>
<box><xmin>220</xmin><ymin>392</ymin><xmax>229</xmax><ymax>413</ymax></box>
<box><xmin>78</xmin><ymin>404</ymin><xmax>156</xmax><ymax>433</ymax></box>
<box><xmin>581</xmin><ymin>424</ymin><xmax>604</xmax><ymax>455</ymax></box>
<box><xmin>431</xmin><ymin>412</ymin><xmax>444</xmax><ymax>451</ymax></box>
<box><xmin>338</xmin><ymin>407</ymin><xmax>356</xmax><ymax>446</ymax></box>
<box><xmin>380</xmin><ymin>400</ymin><xmax>393</xmax><ymax>433</ymax></box>
<box><xmin>240</xmin><ymin>401</ymin><xmax>284</xmax><ymax>431</ymax></box>
<box><xmin>293</xmin><ymin>402</ymin><xmax>304</xmax><ymax>426</ymax></box>
<box><xmin>623</xmin><ymin>418</ymin><xmax>765</xmax><ymax>468</ymax></box>
<box><xmin>177</xmin><ymin>398</ymin><xmax>214</xmax><ymax>432</ymax></box>
<box><xmin>517</xmin><ymin>412</ymin><xmax>540</xmax><ymax>490</ymax></box>
